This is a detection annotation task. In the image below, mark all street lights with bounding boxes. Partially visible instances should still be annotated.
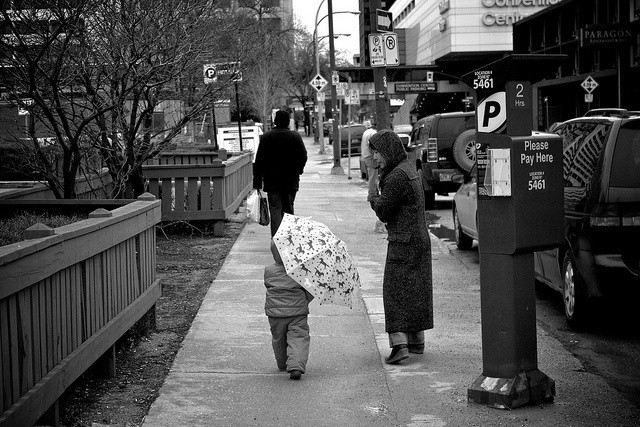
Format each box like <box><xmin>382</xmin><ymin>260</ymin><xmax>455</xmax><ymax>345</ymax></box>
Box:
<box><xmin>314</xmin><ymin>10</ymin><xmax>361</xmax><ymax>144</ymax></box>
<box><xmin>310</xmin><ymin>36</ymin><xmax>339</xmax><ymax>54</ymax></box>
<box><xmin>312</xmin><ymin>33</ymin><xmax>352</xmax><ymax>138</ymax></box>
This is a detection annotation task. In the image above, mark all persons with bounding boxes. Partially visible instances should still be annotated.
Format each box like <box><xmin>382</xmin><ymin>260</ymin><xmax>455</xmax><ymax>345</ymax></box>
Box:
<box><xmin>360</xmin><ymin>129</ymin><xmax>380</xmax><ymax>201</ymax></box>
<box><xmin>302</xmin><ymin>109</ymin><xmax>310</xmax><ymax>135</ymax></box>
<box><xmin>252</xmin><ymin>109</ymin><xmax>307</xmax><ymax>238</ymax></box>
<box><xmin>263</xmin><ymin>240</ymin><xmax>315</xmax><ymax>380</ymax></box>
<box><xmin>368</xmin><ymin>130</ymin><xmax>434</xmax><ymax>364</ymax></box>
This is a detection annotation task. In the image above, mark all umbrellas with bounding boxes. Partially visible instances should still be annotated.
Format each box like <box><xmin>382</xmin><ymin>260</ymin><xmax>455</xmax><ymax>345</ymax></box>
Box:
<box><xmin>272</xmin><ymin>213</ymin><xmax>361</xmax><ymax>309</ymax></box>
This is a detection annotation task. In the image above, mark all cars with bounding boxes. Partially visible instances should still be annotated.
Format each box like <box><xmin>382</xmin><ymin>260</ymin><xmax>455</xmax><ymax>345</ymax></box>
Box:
<box><xmin>323</xmin><ymin>122</ymin><xmax>334</xmax><ymax>136</ymax></box>
<box><xmin>336</xmin><ymin>126</ymin><xmax>367</xmax><ymax>158</ymax></box>
<box><xmin>453</xmin><ymin>159</ymin><xmax>479</xmax><ymax>253</ymax></box>
<box><xmin>329</xmin><ymin>126</ymin><xmax>334</xmax><ymax>144</ymax></box>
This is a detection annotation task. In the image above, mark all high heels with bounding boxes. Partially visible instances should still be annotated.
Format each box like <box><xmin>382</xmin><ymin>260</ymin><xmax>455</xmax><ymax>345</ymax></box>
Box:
<box><xmin>384</xmin><ymin>344</ymin><xmax>408</xmax><ymax>362</ymax></box>
<box><xmin>408</xmin><ymin>342</ymin><xmax>424</xmax><ymax>353</ymax></box>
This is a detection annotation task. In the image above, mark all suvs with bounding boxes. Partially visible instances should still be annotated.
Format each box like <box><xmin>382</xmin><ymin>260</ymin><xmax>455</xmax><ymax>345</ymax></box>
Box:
<box><xmin>410</xmin><ymin>111</ymin><xmax>478</xmax><ymax>210</ymax></box>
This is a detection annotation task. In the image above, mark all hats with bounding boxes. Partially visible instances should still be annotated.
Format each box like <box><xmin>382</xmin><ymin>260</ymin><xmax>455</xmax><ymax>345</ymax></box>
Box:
<box><xmin>275</xmin><ymin>111</ymin><xmax>290</xmax><ymax>123</ymax></box>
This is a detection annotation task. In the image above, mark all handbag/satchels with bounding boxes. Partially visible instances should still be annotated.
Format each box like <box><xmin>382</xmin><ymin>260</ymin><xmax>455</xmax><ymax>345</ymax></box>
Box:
<box><xmin>260</xmin><ymin>193</ymin><xmax>269</xmax><ymax>225</ymax></box>
<box><xmin>251</xmin><ymin>193</ymin><xmax>260</xmax><ymax>223</ymax></box>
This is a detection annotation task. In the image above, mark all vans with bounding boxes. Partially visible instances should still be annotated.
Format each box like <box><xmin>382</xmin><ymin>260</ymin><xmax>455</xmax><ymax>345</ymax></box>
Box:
<box><xmin>534</xmin><ymin>107</ymin><xmax>640</xmax><ymax>324</ymax></box>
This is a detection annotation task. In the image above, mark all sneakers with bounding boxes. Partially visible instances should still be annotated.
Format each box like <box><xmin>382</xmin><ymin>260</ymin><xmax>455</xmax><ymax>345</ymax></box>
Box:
<box><xmin>290</xmin><ymin>370</ymin><xmax>301</xmax><ymax>379</ymax></box>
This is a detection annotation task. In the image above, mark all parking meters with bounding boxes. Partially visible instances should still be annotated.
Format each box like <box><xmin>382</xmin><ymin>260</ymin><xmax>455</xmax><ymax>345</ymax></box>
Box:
<box><xmin>467</xmin><ymin>130</ymin><xmax>566</xmax><ymax>409</ymax></box>
